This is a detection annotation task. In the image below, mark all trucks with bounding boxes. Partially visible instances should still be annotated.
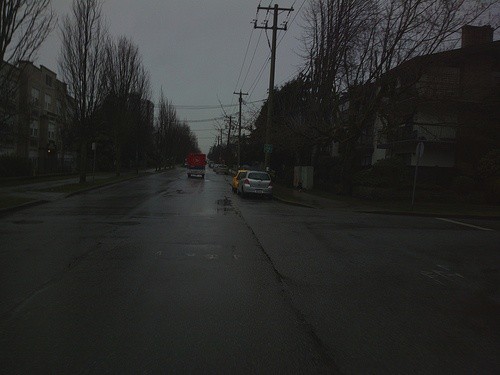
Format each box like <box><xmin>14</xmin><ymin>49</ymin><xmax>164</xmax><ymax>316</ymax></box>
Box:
<box><xmin>186</xmin><ymin>153</ymin><xmax>206</xmax><ymax>178</ymax></box>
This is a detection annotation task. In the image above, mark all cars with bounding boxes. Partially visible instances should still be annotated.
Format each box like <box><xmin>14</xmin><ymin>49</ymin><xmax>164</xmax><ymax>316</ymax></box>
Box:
<box><xmin>238</xmin><ymin>171</ymin><xmax>273</xmax><ymax>199</ymax></box>
<box><xmin>232</xmin><ymin>169</ymin><xmax>250</xmax><ymax>192</ymax></box>
<box><xmin>208</xmin><ymin>160</ymin><xmax>229</xmax><ymax>175</ymax></box>
